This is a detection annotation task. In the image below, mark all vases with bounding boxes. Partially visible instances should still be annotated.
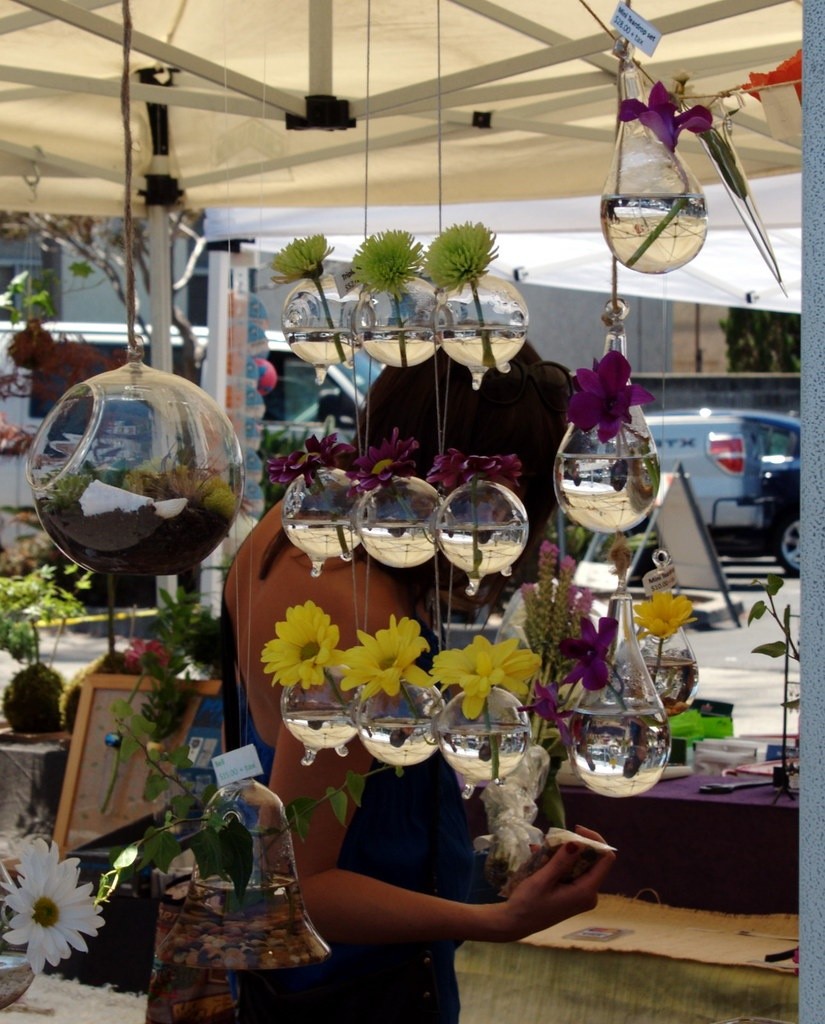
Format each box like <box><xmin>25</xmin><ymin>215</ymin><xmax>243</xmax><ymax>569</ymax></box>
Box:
<box><xmin>0</xmin><ymin>948</ymin><xmax>35</xmax><ymax>1010</ymax></box>
<box><xmin>553</xmin><ymin>331</ymin><xmax>660</xmax><ymax>534</ymax></box>
<box><xmin>436</xmin><ymin>687</ymin><xmax>532</xmax><ymax>800</ymax></box>
<box><xmin>568</xmin><ymin>594</ymin><xmax>668</xmax><ymax>794</ymax></box>
<box><xmin>280</xmin><ymin>667</ymin><xmax>356</xmax><ymax>766</ymax></box>
<box><xmin>280</xmin><ymin>468</ymin><xmax>362</xmax><ymax>578</ymax></box>
<box><xmin>356</xmin><ymin>278</ymin><xmax>443</xmax><ymax>367</ymax></box>
<box><xmin>435</xmin><ymin>479</ymin><xmax>530</xmax><ymax>599</ymax></box>
<box><xmin>355</xmin><ymin>477</ymin><xmax>443</xmax><ymax>568</ymax></box>
<box><xmin>630</xmin><ymin>625</ymin><xmax>698</xmax><ymax>717</ymax></box>
<box><xmin>339</xmin><ymin>613</ymin><xmax>435</xmax><ymax>694</ymax></box>
<box><xmin>532</xmin><ymin>761</ymin><xmax>568</xmax><ymax>841</ymax></box>
<box><xmin>282</xmin><ymin>274</ymin><xmax>361</xmax><ymax>367</ymax></box>
<box><xmin>601</xmin><ymin>30</ymin><xmax>708</xmax><ymax>274</ymax></box>
<box><xmin>437</xmin><ymin>274</ymin><xmax>529</xmax><ymax>392</ymax></box>
<box><xmin>353</xmin><ymin>678</ymin><xmax>447</xmax><ymax>775</ymax></box>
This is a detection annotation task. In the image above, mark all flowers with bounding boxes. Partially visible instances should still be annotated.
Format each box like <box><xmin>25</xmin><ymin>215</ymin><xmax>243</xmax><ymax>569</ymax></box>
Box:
<box><xmin>268</xmin><ymin>233</ymin><xmax>336</xmax><ymax>283</ymax></box>
<box><xmin>350</xmin><ymin>230</ymin><xmax>424</xmax><ymax>304</ymax></box>
<box><xmin>521</xmin><ymin>613</ymin><xmax>625</xmax><ymax>734</ymax></box>
<box><xmin>507</xmin><ymin>539</ymin><xmax>594</xmax><ymax>754</ymax></box>
<box><xmin>424</xmin><ymin>636</ymin><xmax>542</xmax><ymax>720</ymax></box>
<box><xmin>355</xmin><ymin>427</ymin><xmax>421</xmax><ymax>495</ymax></box>
<box><xmin>563</xmin><ymin>350</ymin><xmax>655</xmax><ymax>443</ymax></box>
<box><xmin>265</xmin><ymin>433</ymin><xmax>354</xmax><ymax>489</ymax></box>
<box><xmin>260</xmin><ymin>598</ymin><xmax>338</xmax><ymax>689</ymax></box>
<box><xmin>426</xmin><ymin>448</ymin><xmax>526</xmax><ymax>488</ymax></box>
<box><xmin>423</xmin><ymin>220</ymin><xmax>498</xmax><ymax>294</ymax></box>
<box><xmin>0</xmin><ymin>837</ymin><xmax>105</xmax><ymax>975</ymax></box>
<box><xmin>100</xmin><ymin>643</ymin><xmax>170</xmax><ymax>817</ymax></box>
<box><xmin>619</xmin><ymin>81</ymin><xmax>716</xmax><ymax>152</ymax></box>
<box><xmin>632</xmin><ymin>585</ymin><xmax>691</xmax><ymax>643</ymax></box>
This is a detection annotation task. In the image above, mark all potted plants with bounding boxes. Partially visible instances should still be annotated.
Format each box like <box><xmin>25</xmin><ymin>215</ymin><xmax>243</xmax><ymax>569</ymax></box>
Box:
<box><xmin>91</xmin><ymin>588</ymin><xmax>404</xmax><ymax>1024</ymax></box>
<box><xmin>26</xmin><ymin>357</ymin><xmax>244</xmax><ymax>577</ymax></box>
<box><xmin>0</xmin><ymin>565</ymin><xmax>93</xmax><ymax>735</ymax></box>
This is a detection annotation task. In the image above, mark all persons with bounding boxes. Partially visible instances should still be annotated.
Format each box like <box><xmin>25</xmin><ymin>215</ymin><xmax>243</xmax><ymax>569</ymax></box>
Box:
<box><xmin>216</xmin><ymin>323</ymin><xmax>616</xmax><ymax>1024</ymax></box>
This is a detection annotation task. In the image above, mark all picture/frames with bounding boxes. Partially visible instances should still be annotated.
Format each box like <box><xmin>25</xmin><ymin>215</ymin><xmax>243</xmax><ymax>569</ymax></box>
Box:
<box><xmin>49</xmin><ymin>675</ymin><xmax>225</xmax><ymax>865</ymax></box>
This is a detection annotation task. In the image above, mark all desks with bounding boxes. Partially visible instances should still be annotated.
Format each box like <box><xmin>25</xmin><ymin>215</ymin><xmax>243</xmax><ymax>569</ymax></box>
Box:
<box><xmin>560</xmin><ymin>776</ymin><xmax>800</xmax><ymax>919</ymax></box>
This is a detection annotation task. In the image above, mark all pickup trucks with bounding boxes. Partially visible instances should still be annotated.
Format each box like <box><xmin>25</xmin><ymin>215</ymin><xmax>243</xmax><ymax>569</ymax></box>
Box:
<box><xmin>640</xmin><ymin>416</ymin><xmax>764</xmax><ymax>528</ymax></box>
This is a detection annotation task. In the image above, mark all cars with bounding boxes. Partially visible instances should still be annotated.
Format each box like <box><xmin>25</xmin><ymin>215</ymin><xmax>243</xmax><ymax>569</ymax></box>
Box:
<box><xmin>634</xmin><ymin>410</ymin><xmax>801</xmax><ymax>576</ymax></box>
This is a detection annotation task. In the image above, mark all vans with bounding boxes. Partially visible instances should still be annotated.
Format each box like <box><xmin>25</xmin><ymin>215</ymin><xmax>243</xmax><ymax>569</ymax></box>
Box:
<box><xmin>0</xmin><ymin>319</ymin><xmax>385</xmax><ymax>548</ymax></box>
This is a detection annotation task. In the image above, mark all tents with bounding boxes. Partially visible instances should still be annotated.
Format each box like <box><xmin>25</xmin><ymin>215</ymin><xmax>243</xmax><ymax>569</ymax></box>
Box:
<box><xmin>199</xmin><ymin>171</ymin><xmax>801</xmax><ymax>622</ymax></box>
